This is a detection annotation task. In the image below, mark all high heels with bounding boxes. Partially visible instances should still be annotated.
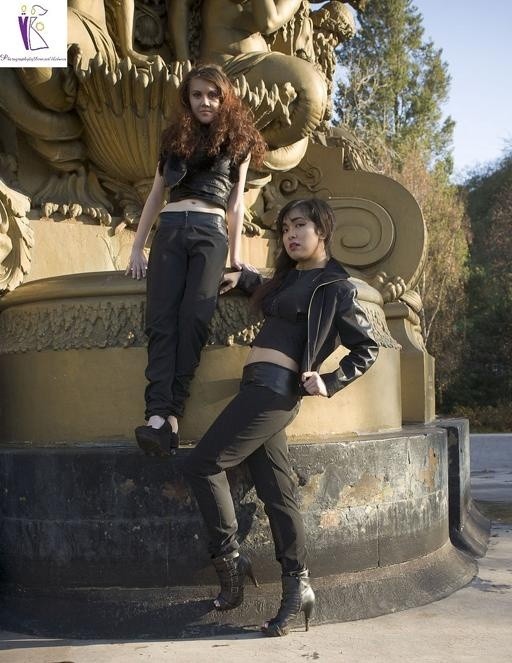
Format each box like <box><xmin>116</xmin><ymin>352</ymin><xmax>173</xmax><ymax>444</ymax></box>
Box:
<box><xmin>261</xmin><ymin>569</ymin><xmax>315</xmax><ymax>636</ymax></box>
<box><xmin>209</xmin><ymin>549</ymin><xmax>259</xmax><ymax>610</ymax></box>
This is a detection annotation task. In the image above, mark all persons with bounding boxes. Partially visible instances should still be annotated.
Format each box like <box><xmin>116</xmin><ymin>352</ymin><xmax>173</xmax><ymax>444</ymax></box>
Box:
<box><xmin>123</xmin><ymin>62</ymin><xmax>266</xmax><ymax>454</ymax></box>
<box><xmin>67</xmin><ymin>0</ymin><xmax>365</xmax><ymax>172</ymax></box>
<box><xmin>183</xmin><ymin>196</ymin><xmax>380</xmax><ymax>637</ymax></box>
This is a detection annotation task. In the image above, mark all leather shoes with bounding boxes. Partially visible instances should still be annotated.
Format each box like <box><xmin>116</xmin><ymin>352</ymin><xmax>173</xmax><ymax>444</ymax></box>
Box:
<box><xmin>135</xmin><ymin>420</ymin><xmax>172</xmax><ymax>456</ymax></box>
<box><xmin>170</xmin><ymin>431</ymin><xmax>180</xmax><ymax>447</ymax></box>
